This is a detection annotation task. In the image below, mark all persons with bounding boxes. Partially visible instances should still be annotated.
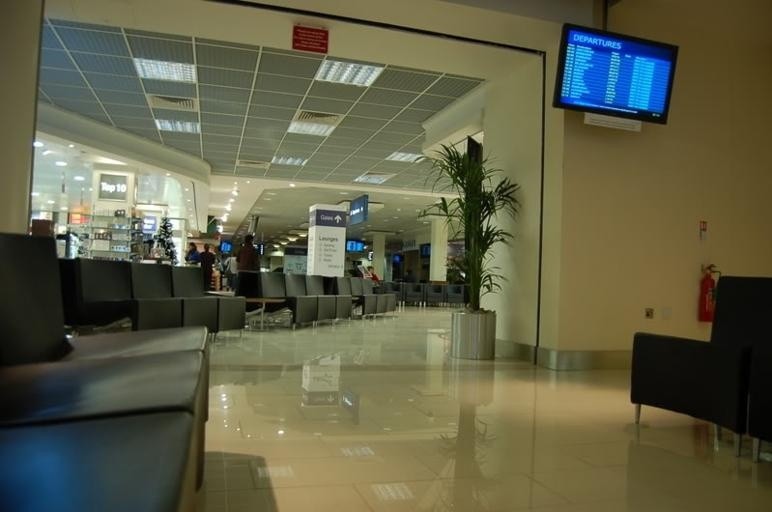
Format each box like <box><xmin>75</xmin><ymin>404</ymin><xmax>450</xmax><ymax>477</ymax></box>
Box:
<box><xmin>184</xmin><ymin>243</ymin><xmax>202</xmax><ymax>266</ymax></box>
<box><xmin>349</xmin><ymin>261</ymin><xmax>363</xmax><ymax>278</ymax></box>
<box><xmin>200</xmin><ymin>243</ymin><xmax>215</xmax><ymax>291</ymax></box>
<box><xmin>367</xmin><ymin>266</ymin><xmax>378</xmax><ymax>282</ymax></box>
<box><xmin>70</xmin><ymin>231</ymin><xmax>89</xmax><ymax>259</ymax></box>
<box><xmin>236</xmin><ymin>235</ymin><xmax>259</xmax><ymax>271</ymax></box>
<box><xmin>213</xmin><ymin>241</ymin><xmax>245</xmax><ymax>292</ymax></box>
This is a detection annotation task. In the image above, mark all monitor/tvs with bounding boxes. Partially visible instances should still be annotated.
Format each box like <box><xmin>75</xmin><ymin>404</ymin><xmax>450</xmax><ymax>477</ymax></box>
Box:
<box><xmin>553</xmin><ymin>22</ymin><xmax>679</xmax><ymax>126</ymax></box>
<box><xmin>220</xmin><ymin>238</ymin><xmax>432</xmax><ymax>263</ymax></box>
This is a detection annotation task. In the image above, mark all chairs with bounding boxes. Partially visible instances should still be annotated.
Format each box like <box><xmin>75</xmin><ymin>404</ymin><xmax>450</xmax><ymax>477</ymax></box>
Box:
<box><xmin>630</xmin><ymin>276</ymin><xmax>772</xmax><ymax>462</ymax></box>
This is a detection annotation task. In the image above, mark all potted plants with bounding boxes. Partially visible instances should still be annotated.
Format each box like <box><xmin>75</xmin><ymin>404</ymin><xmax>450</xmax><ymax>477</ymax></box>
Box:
<box><xmin>417</xmin><ymin>141</ymin><xmax>521</xmax><ymax>361</ymax></box>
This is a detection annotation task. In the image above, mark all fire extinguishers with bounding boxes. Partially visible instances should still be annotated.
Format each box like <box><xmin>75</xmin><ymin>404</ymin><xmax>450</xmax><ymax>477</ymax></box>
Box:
<box><xmin>699</xmin><ymin>264</ymin><xmax>721</xmax><ymax>322</ymax></box>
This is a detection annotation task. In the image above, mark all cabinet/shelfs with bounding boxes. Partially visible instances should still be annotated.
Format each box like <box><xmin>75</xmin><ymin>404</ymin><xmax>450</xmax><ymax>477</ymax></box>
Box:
<box><xmin>91</xmin><ymin>215</ymin><xmax>131</xmax><ymax>262</ymax></box>
